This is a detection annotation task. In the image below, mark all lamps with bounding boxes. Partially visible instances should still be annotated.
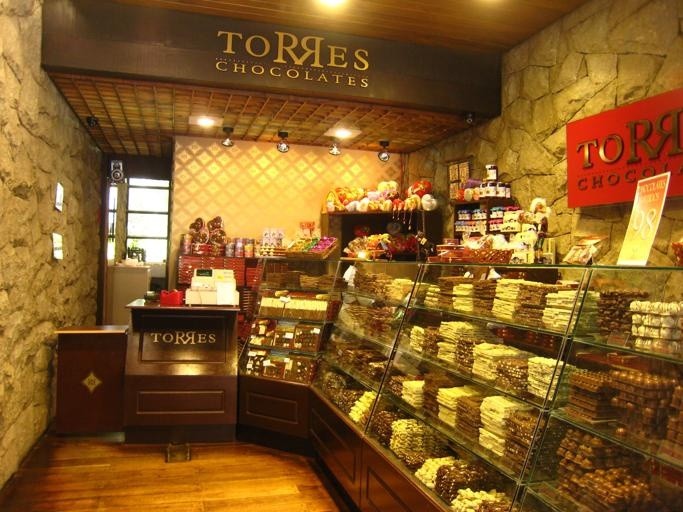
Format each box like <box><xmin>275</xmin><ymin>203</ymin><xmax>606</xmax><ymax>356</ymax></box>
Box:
<box><xmin>328</xmin><ymin>137</ymin><xmax>342</xmax><ymax>156</ymax></box>
<box><xmin>275</xmin><ymin>131</ymin><xmax>291</xmax><ymax>153</ymax></box>
<box><xmin>220</xmin><ymin>112</ymin><xmax>238</xmax><ymax>147</ymax></box>
<box><xmin>377</xmin><ymin>141</ymin><xmax>391</xmax><ymax>161</ymax></box>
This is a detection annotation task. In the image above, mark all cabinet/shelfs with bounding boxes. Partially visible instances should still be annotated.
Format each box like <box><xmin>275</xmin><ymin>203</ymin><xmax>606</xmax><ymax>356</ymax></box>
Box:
<box><xmin>451</xmin><ymin>196</ymin><xmax>520</xmax><ymax>238</ymax></box>
<box><xmin>514</xmin><ymin>267</ymin><xmax>683</xmax><ymax>509</ymax></box>
<box><xmin>53</xmin><ymin>322</ymin><xmax>130</xmax><ymax>438</ymax></box>
<box><xmin>236</xmin><ymin>258</ymin><xmax>343</xmax><ymax>440</ymax></box>
<box><xmin>359</xmin><ymin>264</ymin><xmax>590</xmax><ymax>509</ymax></box>
<box><xmin>305</xmin><ymin>260</ymin><xmax>421</xmax><ymax>509</ymax></box>
